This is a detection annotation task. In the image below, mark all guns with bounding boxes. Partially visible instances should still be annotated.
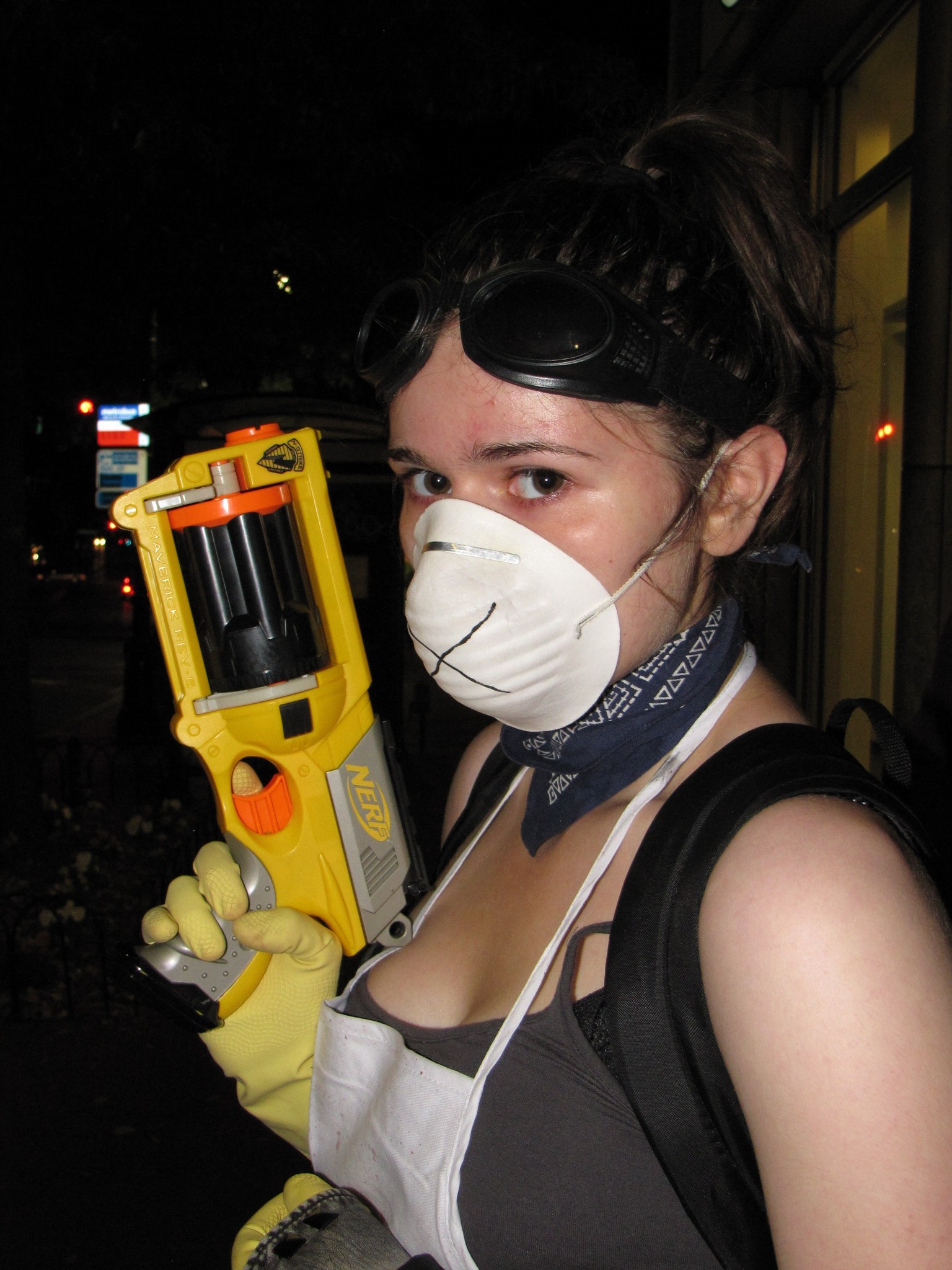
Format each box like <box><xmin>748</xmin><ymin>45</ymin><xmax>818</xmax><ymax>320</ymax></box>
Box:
<box><xmin>109</xmin><ymin>424</ymin><xmax>426</xmax><ymax>1029</ymax></box>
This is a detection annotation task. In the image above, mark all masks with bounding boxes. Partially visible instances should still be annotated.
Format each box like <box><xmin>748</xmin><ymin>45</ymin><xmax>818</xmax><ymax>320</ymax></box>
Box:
<box><xmin>404</xmin><ymin>499</ymin><xmax>621</xmax><ymax>731</ymax></box>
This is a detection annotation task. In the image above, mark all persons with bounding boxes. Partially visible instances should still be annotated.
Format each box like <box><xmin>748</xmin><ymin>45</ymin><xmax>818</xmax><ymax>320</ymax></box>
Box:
<box><xmin>145</xmin><ymin>109</ymin><xmax>952</xmax><ymax>1270</ymax></box>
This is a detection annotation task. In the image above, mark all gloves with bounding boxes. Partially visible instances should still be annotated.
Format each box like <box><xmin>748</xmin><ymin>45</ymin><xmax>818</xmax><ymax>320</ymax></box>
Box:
<box><xmin>231</xmin><ymin>1173</ymin><xmax>372</xmax><ymax>1270</ymax></box>
<box><xmin>141</xmin><ymin>841</ymin><xmax>342</xmax><ymax>1160</ymax></box>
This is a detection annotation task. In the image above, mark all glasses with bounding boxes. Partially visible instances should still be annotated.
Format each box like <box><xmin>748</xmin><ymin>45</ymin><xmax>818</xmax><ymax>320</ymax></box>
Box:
<box><xmin>354</xmin><ymin>260</ymin><xmax>661</xmax><ymax>404</ymax></box>
